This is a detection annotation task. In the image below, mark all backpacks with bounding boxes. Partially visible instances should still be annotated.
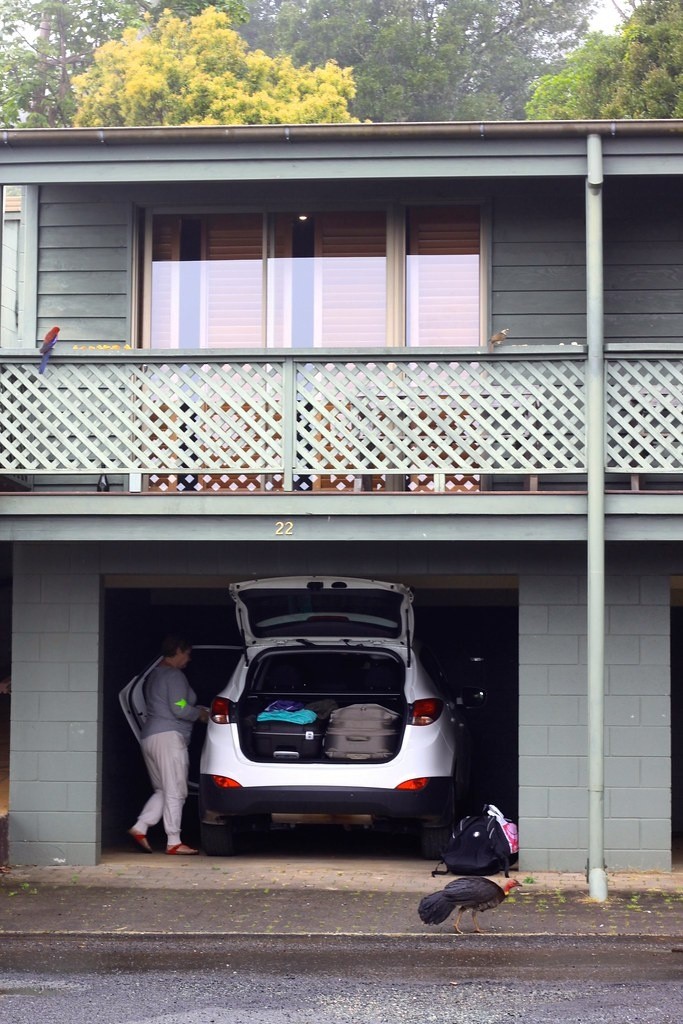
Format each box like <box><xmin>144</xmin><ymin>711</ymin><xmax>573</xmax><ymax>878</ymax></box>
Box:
<box><xmin>431</xmin><ymin>814</ymin><xmax>511</xmax><ymax>879</ymax></box>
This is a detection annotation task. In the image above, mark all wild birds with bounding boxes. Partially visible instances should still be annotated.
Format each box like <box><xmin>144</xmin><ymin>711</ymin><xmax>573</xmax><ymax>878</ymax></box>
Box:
<box><xmin>418</xmin><ymin>878</ymin><xmax>522</xmax><ymax>935</ymax></box>
<box><xmin>38</xmin><ymin>327</ymin><xmax>61</xmax><ymax>354</ymax></box>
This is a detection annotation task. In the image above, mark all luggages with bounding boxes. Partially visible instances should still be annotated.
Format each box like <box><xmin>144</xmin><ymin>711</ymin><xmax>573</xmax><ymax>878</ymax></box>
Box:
<box><xmin>250</xmin><ymin>710</ymin><xmax>323</xmax><ymax>762</ymax></box>
<box><xmin>324</xmin><ymin>702</ymin><xmax>402</xmax><ymax>762</ymax></box>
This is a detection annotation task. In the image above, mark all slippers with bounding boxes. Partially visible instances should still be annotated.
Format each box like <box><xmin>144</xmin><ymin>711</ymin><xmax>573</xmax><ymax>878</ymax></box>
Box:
<box><xmin>164</xmin><ymin>841</ymin><xmax>198</xmax><ymax>854</ymax></box>
<box><xmin>128</xmin><ymin>826</ymin><xmax>152</xmax><ymax>853</ymax></box>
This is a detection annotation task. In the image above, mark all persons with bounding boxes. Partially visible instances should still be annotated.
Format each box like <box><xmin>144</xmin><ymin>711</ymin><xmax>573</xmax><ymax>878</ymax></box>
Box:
<box><xmin>127</xmin><ymin>635</ymin><xmax>211</xmax><ymax>855</ymax></box>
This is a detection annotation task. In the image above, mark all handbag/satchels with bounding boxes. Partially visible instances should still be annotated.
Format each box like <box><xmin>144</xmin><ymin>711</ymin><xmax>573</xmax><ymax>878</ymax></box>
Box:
<box><xmin>486</xmin><ymin>803</ymin><xmax>519</xmax><ymax>854</ymax></box>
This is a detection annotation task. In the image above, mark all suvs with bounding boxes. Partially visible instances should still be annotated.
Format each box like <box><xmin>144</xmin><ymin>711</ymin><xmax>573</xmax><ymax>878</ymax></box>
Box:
<box><xmin>115</xmin><ymin>574</ymin><xmax>491</xmax><ymax>857</ymax></box>
